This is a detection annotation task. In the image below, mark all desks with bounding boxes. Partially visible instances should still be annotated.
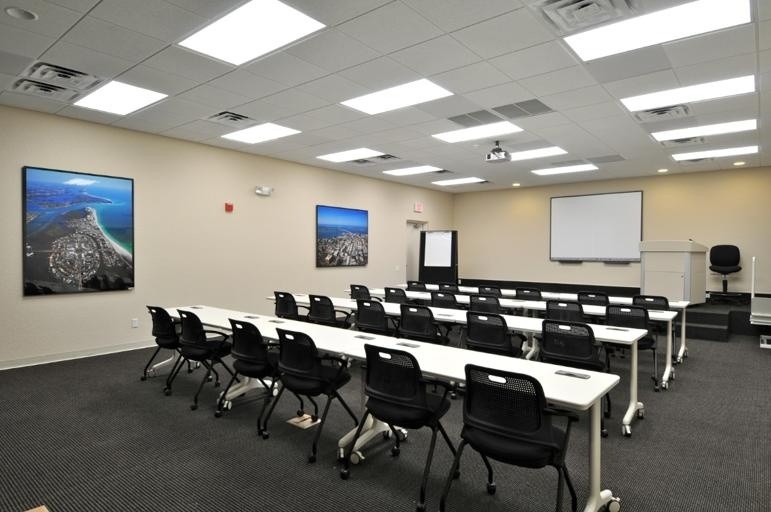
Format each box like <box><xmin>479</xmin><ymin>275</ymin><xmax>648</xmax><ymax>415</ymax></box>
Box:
<box><xmin>677</xmin><ymin>300</ymin><xmax>691</xmax><ymax>364</ymax></box>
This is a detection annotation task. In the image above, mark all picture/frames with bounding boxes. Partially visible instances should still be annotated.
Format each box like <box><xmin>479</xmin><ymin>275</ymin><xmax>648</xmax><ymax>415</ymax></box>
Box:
<box><xmin>22</xmin><ymin>165</ymin><xmax>136</xmax><ymax>295</ymax></box>
<box><xmin>315</xmin><ymin>204</ymin><xmax>368</xmax><ymax>266</ymax></box>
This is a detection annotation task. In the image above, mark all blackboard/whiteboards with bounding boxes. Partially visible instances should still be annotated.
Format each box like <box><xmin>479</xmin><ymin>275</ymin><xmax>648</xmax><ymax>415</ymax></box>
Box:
<box><xmin>548</xmin><ymin>191</ymin><xmax>644</xmax><ymax>263</ymax></box>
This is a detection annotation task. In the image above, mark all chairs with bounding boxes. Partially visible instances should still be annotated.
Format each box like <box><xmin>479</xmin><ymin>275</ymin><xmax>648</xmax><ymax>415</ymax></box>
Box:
<box><xmin>707</xmin><ymin>244</ymin><xmax>750</xmax><ymax>307</ymax></box>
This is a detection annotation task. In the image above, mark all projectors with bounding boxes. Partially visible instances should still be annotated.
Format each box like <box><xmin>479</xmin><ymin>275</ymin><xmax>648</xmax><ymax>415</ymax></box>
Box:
<box><xmin>485</xmin><ymin>148</ymin><xmax>511</xmax><ymax>162</ymax></box>
